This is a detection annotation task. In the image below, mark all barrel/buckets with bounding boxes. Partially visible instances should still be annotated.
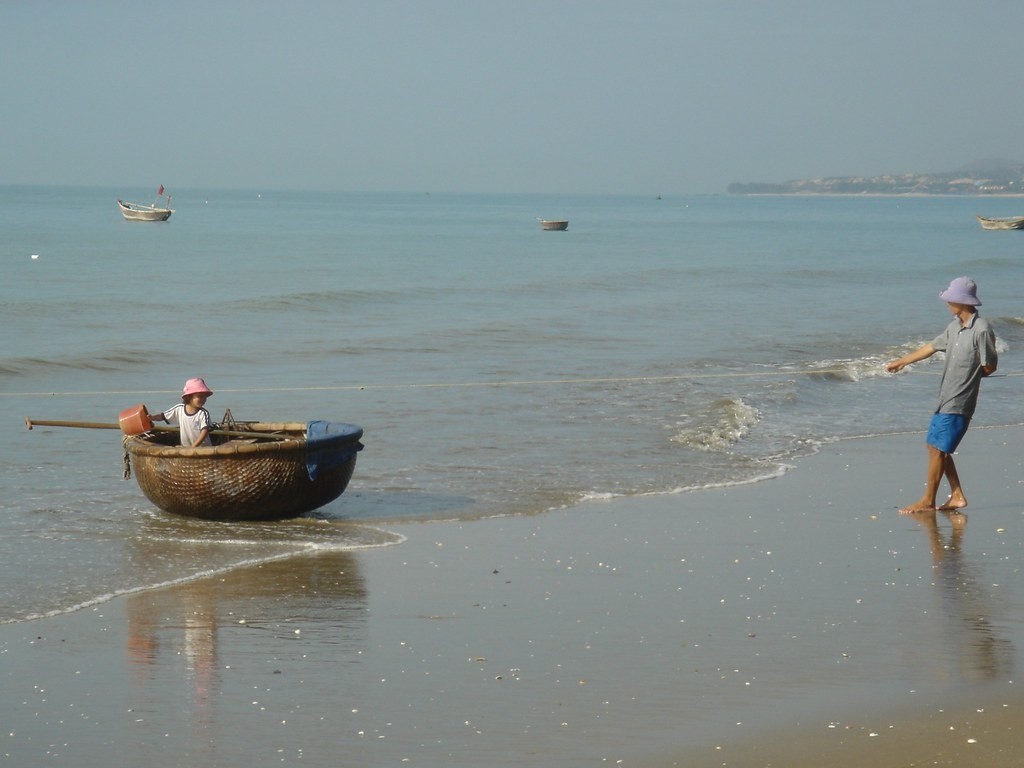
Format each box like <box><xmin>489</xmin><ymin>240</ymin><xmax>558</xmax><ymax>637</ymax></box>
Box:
<box><xmin>118</xmin><ymin>403</ymin><xmax>155</xmax><ymax>436</ymax></box>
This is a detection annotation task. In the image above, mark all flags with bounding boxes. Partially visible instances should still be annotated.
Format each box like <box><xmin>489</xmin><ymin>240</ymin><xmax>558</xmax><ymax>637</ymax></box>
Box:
<box><xmin>158</xmin><ymin>185</ymin><xmax>164</xmax><ymax>196</ymax></box>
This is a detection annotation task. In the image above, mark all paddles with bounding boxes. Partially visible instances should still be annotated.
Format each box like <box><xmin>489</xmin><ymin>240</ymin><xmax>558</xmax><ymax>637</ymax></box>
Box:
<box><xmin>24</xmin><ymin>417</ymin><xmax>300</xmax><ymax>442</ymax></box>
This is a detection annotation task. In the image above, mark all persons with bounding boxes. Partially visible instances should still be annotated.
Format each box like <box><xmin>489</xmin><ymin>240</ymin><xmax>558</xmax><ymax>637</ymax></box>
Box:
<box><xmin>147</xmin><ymin>377</ymin><xmax>212</xmax><ymax>447</ymax></box>
<box><xmin>886</xmin><ymin>276</ymin><xmax>998</xmax><ymax>514</ymax></box>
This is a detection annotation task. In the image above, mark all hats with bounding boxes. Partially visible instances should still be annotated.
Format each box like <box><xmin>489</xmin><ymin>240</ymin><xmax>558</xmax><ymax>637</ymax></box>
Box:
<box><xmin>939</xmin><ymin>275</ymin><xmax>982</xmax><ymax>306</ymax></box>
<box><xmin>182</xmin><ymin>377</ymin><xmax>213</xmax><ymax>398</ymax></box>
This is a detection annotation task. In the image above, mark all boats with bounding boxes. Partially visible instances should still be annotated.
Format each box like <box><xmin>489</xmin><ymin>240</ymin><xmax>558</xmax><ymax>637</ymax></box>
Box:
<box><xmin>539</xmin><ymin>220</ymin><xmax>569</xmax><ymax>230</ymax></box>
<box><xmin>975</xmin><ymin>214</ymin><xmax>1024</xmax><ymax>230</ymax></box>
<box><xmin>116</xmin><ymin>198</ymin><xmax>171</xmax><ymax>220</ymax></box>
<box><xmin>122</xmin><ymin>408</ymin><xmax>362</xmax><ymax>517</ymax></box>
